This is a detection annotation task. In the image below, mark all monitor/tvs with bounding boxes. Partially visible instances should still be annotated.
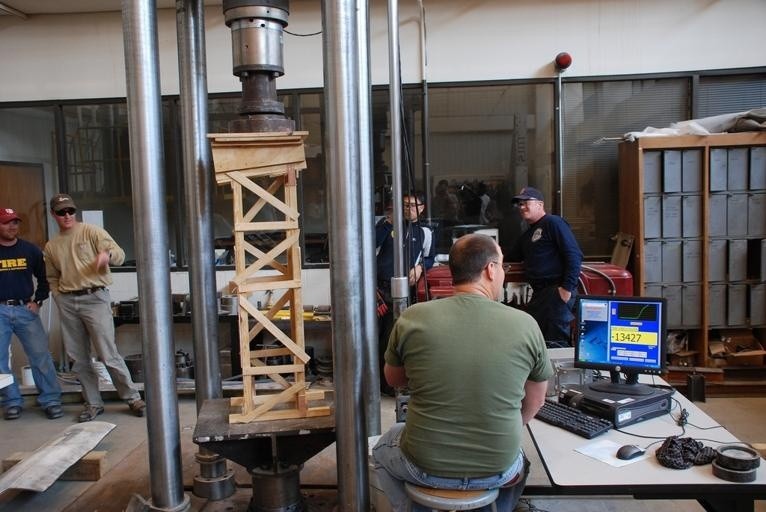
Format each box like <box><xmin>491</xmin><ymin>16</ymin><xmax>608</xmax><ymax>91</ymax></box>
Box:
<box><xmin>574</xmin><ymin>294</ymin><xmax>667</xmax><ymax>395</ymax></box>
<box><xmin>534</xmin><ymin>397</ymin><xmax>613</xmax><ymax>439</ymax></box>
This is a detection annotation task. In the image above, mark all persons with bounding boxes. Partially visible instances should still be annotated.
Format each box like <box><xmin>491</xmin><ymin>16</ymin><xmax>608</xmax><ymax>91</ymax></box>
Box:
<box><xmin>430</xmin><ymin>178</ymin><xmax>504</xmax><ymax>240</ymax></box>
<box><xmin>372</xmin><ymin>192</ymin><xmax>437</xmax><ymax>396</ymax></box>
<box><xmin>45</xmin><ymin>195</ymin><xmax>151</xmax><ymax>423</ymax></box>
<box><xmin>372</xmin><ymin>232</ymin><xmax>549</xmax><ymax>512</ymax></box>
<box><xmin>1</xmin><ymin>209</ymin><xmax>65</xmax><ymax>420</ymax></box>
<box><xmin>500</xmin><ymin>187</ymin><xmax>580</xmax><ymax>353</ymax></box>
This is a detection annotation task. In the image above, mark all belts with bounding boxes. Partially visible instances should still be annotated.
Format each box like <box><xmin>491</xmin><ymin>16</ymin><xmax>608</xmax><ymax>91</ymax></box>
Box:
<box><xmin>0</xmin><ymin>296</ymin><xmax>31</xmax><ymax>306</ymax></box>
<box><xmin>58</xmin><ymin>285</ymin><xmax>105</xmax><ymax>296</ymax></box>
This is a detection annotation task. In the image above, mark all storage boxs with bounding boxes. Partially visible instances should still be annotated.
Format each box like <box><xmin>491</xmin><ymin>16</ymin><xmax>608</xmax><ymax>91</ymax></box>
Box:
<box><xmin>710</xmin><ymin>331</ymin><xmax>766</xmax><ymax>367</ymax></box>
<box><xmin>669</xmin><ymin>350</ymin><xmax>700</xmax><ymax>367</ymax></box>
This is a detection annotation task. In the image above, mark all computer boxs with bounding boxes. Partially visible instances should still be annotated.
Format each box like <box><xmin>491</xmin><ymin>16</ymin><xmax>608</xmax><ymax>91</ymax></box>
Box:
<box><xmin>558</xmin><ymin>383</ymin><xmax>671</xmax><ymax>429</ymax></box>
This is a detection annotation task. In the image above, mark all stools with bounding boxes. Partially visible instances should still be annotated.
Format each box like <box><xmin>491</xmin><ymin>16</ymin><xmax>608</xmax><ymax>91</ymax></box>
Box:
<box><xmin>403</xmin><ymin>480</ymin><xmax>500</xmax><ymax>512</ymax></box>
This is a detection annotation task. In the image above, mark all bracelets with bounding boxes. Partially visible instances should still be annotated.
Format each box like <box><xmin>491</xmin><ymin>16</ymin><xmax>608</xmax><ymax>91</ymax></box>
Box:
<box><xmin>106</xmin><ymin>250</ymin><xmax>114</xmax><ymax>262</ymax></box>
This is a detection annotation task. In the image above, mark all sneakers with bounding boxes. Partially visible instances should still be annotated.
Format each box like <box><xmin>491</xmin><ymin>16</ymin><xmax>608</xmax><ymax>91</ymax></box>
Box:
<box><xmin>3</xmin><ymin>406</ymin><xmax>23</xmax><ymax>420</ymax></box>
<box><xmin>128</xmin><ymin>398</ymin><xmax>146</xmax><ymax>414</ymax></box>
<box><xmin>79</xmin><ymin>406</ymin><xmax>104</xmax><ymax>422</ymax></box>
<box><xmin>45</xmin><ymin>404</ymin><xmax>64</xmax><ymax>419</ymax></box>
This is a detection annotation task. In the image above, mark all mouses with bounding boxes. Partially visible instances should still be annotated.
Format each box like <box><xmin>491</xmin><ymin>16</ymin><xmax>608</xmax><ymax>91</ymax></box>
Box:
<box><xmin>616</xmin><ymin>445</ymin><xmax>645</xmax><ymax>460</ymax></box>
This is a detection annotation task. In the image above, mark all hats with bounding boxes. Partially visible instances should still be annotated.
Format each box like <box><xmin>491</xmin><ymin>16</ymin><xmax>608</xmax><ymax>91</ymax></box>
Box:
<box><xmin>0</xmin><ymin>207</ymin><xmax>23</xmax><ymax>224</ymax></box>
<box><xmin>511</xmin><ymin>186</ymin><xmax>544</xmax><ymax>204</ymax></box>
<box><xmin>50</xmin><ymin>193</ymin><xmax>77</xmax><ymax>212</ymax></box>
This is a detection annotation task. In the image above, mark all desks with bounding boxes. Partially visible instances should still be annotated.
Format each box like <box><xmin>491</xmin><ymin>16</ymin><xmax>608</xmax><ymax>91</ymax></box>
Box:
<box><xmin>526</xmin><ymin>345</ymin><xmax>766</xmax><ymax>511</ymax></box>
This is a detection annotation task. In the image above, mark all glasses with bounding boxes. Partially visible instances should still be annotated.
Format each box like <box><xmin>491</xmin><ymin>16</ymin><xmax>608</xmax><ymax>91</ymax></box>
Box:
<box><xmin>484</xmin><ymin>260</ymin><xmax>512</xmax><ymax>276</ymax></box>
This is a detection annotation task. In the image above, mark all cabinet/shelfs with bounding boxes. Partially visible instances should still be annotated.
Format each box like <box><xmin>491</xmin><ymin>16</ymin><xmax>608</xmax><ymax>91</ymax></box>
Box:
<box><xmin>110</xmin><ymin>311</ymin><xmax>333</xmax><ymax>401</ymax></box>
<box><xmin>616</xmin><ymin>130</ymin><xmax>766</xmax><ymax>396</ymax></box>
